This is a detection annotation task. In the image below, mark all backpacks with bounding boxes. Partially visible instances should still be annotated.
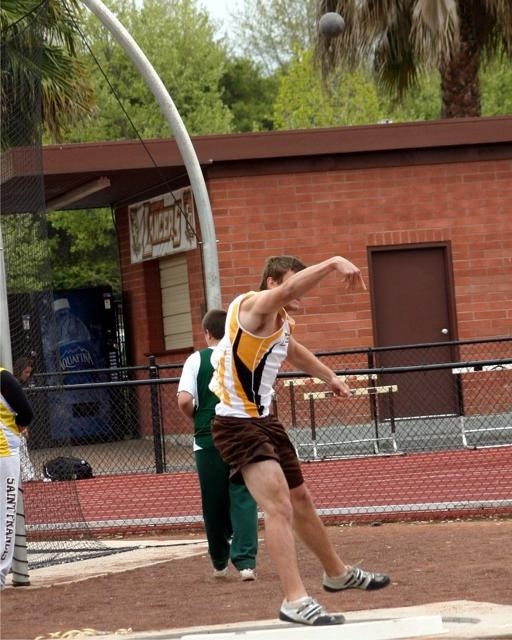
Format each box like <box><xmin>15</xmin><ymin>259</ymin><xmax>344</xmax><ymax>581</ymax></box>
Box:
<box><xmin>40</xmin><ymin>456</ymin><xmax>93</xmax><ymax>481</ymax></box>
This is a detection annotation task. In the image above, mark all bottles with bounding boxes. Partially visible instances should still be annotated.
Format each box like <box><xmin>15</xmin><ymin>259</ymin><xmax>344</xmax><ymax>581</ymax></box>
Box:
<box><xmin>54</xmin><ymin>298</ymin><xmax>98</xmax><ymax>390</ymax></box>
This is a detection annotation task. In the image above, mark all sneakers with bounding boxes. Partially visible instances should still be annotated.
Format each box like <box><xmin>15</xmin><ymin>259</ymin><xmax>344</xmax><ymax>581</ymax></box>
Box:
<box><xmin>322</xmin><ymin>565</ymin><xmax>391</xmax><ymax>593</ymax></box>
<box><xmin>240</xmin><ymin>568</ymin><xmax>257</xmax><ymax>581</ymax></box>
<box><xmin>279</xmin><ymin>595</ymin><xmax>345</xmax><ymax>625</ymax></box>
<box><xmin>213</xmin><ymin>567</ymin><xmax>229</xmax><ymax>578</ymax></box>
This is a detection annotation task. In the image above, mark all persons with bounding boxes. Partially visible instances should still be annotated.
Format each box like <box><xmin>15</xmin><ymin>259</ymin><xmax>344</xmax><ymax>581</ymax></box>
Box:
<box><xmin>177</xmin><ymin>309</ymin><xmax>258</xmax><ymax>582</ymax></box>
<box><xmin>0</xmin><ymin>367</ymin><xmax>35</xmax><ymax>591</ymax></box>
<box><xmin>12</xmin><ymin>357</ymin><xmax>40</xmax><ymax>483</ymax></box>
<box><xmin>207</xmin><ymin>255</ymin><xmax>390</xmax><ymax>625</ymax></box>
